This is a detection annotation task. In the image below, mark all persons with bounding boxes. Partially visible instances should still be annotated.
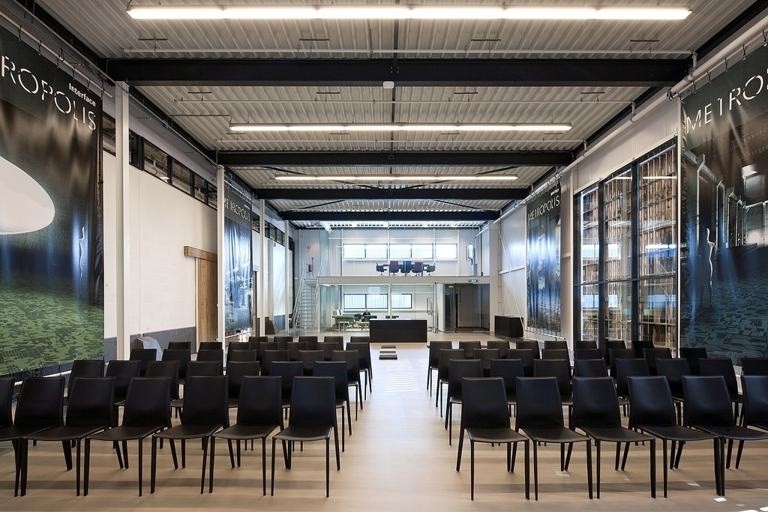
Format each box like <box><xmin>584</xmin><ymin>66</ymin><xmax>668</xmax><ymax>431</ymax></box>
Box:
<box><xmin>362</xmin><ymin>310</ymin><xmax>371</xmax><ymax>322</ymax></box>
<box><xmin>697</xmin><ymin>227</ymin><xmax>715</xmax><ymax>313</ymax></box>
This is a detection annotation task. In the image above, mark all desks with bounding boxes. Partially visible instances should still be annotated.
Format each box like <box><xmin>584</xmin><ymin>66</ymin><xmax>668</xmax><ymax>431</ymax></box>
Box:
<box><xmin>494</xmin><ymin>315</ymin><xmax>523</xmax><ymax>338</ymax></box>
<box><xmin>369</xmin><ymin>318</ymin><xmax>427</xmax><ymax>343</ymax></box>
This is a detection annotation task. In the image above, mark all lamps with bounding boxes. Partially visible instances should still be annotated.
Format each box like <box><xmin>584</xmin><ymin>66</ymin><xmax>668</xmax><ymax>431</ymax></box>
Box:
<box><xmin>274</xmin><ymin>142</ymin><xmax>519</xmax><ymax>184</ymax></box>
<box><xmin>227</xmin><ymin>103</ymin><xmax>574</xmax><ymax>136</ymax></box>
<box><xmin>125</xmin><ymin>0</ymin><xmax>693</xmax><ymax>24</ymax></box>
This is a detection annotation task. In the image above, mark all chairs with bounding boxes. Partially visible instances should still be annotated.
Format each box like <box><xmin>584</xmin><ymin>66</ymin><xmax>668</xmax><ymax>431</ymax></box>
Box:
<box><xmin>0</xmin><ymin>336</ymin><xmax>373</xmax><ymax>500</ymax></box>
<box><xmin>332</xmin><ymin>308</ymin><xmax>398</xmax><ymax>332</ymax></box>
<box><xmin>374</xmin><ymin>261</ymin><xmax>435</xmax><ymax>277</ymax></box>
<box><xmin>427</xmin><ymin>339</ymin><xmax>768</xmax><ymax>501</ymax></box>
